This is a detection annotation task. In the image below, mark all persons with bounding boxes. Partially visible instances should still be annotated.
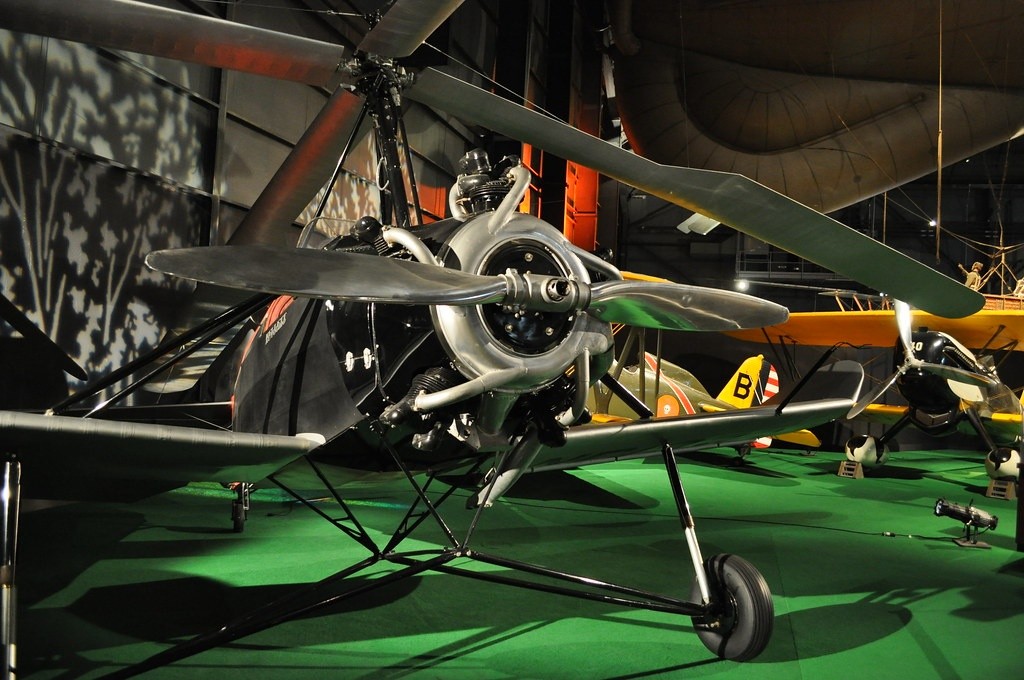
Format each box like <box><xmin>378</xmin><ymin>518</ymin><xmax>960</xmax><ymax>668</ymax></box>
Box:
<box><xmin>956</xmin><ymin>262</ymin><xmax>983</xmax><ymax>291</ymax></box>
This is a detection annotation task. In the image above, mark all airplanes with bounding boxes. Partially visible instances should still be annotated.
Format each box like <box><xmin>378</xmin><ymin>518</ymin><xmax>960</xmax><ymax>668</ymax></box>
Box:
<box><xmin>2</xmin><ymin>2</ymin><xmax>1024</xmax><ymax>663</ymax></box>
